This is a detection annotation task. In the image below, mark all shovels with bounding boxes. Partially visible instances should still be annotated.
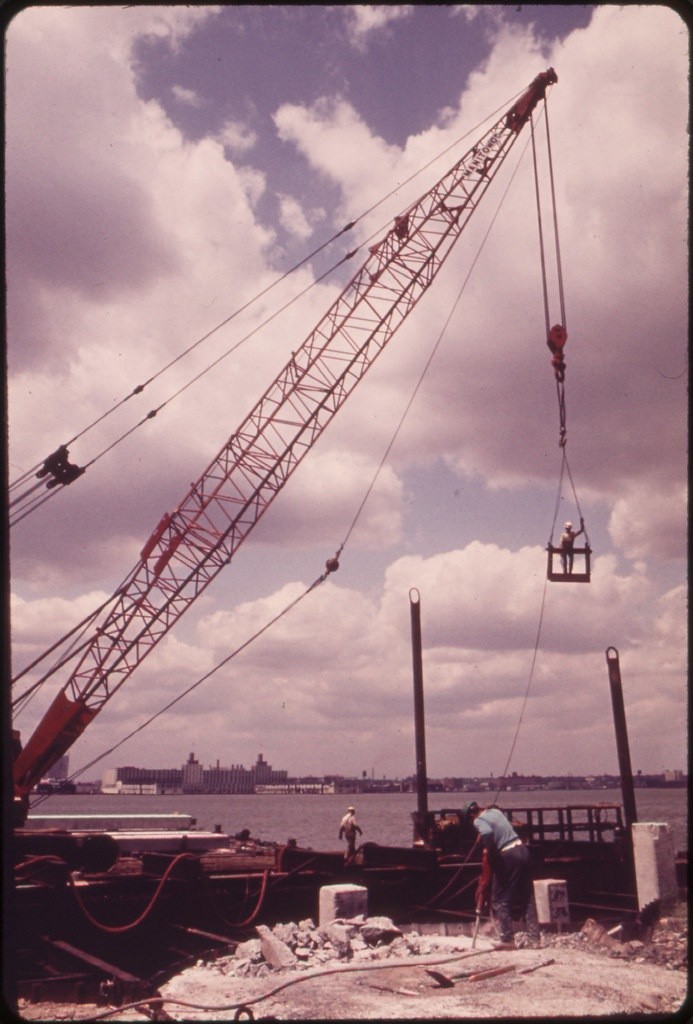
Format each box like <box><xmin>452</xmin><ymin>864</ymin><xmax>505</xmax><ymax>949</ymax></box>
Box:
<box><xmin>425</xmin><ymin>965</ymin><xmax>502</xmax><ymax>987</ymax></box>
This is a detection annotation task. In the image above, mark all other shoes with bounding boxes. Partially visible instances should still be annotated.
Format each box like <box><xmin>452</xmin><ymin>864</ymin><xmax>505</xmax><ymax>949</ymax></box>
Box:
<box><xmin>518</xmin><ymin>940</ymin><xmax>541</xmax><ymax>949</ymax></box>
<box><xmin>491</xmin><ymin>939</ymin><xmax>515</xmax><ymax>949</ymax></box>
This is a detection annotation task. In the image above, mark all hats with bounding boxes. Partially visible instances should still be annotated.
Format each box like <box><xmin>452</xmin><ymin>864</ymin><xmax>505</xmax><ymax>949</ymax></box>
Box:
<box><xmin>348</xmin><ymin>806</ymin><xmax>354</xmax><ymax>810</ymax></box>
<box><xmin>462</xmin><ymin>800</ymin><xmax>476</xmax><ymax>817</ymax></box>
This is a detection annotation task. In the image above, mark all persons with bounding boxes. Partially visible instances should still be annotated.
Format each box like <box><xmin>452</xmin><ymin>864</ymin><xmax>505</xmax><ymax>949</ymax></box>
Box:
<box><xmin>463</xmin><ymin>800</ymin><xmax>530</xmax><ymax>940</ymax></box>
<box><xmin>339</xmin><ymin>807</ymin><xmax>364</xmax><ymax>859</ymax></box>
<box><xmin>559</xmin><ymin>518</ymin><xmax>584</xmax><ymax>575</ymax></box>
